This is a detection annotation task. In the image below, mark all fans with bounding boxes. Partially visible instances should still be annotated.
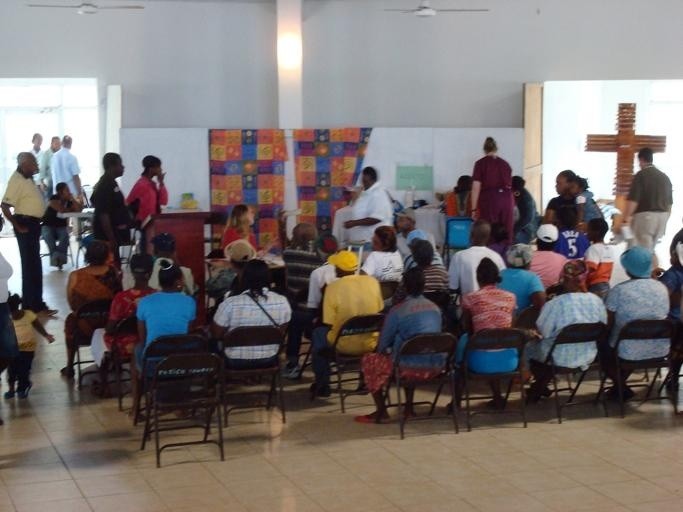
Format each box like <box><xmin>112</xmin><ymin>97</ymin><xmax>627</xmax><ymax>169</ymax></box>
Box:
<box><xmin>384</xmin><ymin>0</ymin><xmax>489</xmax><ymax>17</ymax></box>
<box><xmin>27</xmin><ymin>2</ymin><xmax>144</xmax><ymax>15</ymax></box>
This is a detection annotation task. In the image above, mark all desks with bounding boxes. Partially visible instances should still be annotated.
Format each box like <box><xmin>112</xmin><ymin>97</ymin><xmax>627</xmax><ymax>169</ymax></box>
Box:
<box><xmin>57</xmin><ymin>207</ymin><xmax>95</xmax><ymax>269</ymax></box>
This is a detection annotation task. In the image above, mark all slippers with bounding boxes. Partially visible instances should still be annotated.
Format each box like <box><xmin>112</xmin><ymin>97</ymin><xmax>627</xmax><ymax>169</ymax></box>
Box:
<box><xmin>355</xmin><ymin>414</ymin><xmax>380</xmax><ymax>423</ymax></box>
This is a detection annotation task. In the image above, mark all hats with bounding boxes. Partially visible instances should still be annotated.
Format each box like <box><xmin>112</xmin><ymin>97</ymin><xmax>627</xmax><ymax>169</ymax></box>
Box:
<box><xmin>536</xmin><ymin>223</ymin><xmax>559</xmax><ymax>243</ymax></box>
<box><xmin>223</xmin><ymin>239</ymin><xmax>257</xmax><ymax>262</ymax></box>
<box><xmin>327</xmin><ymin>250</ymin><xmax>358</xmax><ymax>271</ymax></box>
<box><xmin>621</xmin><ymin>246</ymin><xmax>652</xmax><ymax>278</ymax></box>
<box><xmin>394</xmin><ymin>208</ymin><xmax>415</xmax><ymax>221</ymax></box>
<box><xmin>406</xmin><ymin>230</ymin><xmax>427</xmax><ymax>247</ymax></box>
<box><xmin>505</xmin><ymin>243</ymin><xmax>532</xmax><ymax>267</ymax></box>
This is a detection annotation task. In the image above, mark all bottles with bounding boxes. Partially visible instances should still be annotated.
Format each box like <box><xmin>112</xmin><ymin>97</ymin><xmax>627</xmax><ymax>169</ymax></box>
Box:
<box><xmin>406</xmin><ymin>187</ymin><xmax>413</xmax><ymax>207</ymax></box>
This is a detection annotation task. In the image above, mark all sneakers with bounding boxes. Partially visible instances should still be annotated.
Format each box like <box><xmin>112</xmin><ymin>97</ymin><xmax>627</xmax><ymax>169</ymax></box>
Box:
<box><xmin>279</xmin><ymin>368</ymin><xmax>298</xmax><ymax>379</ymax></box>
<box><xmin>61</xmin><ymin>367</ymin><xmax>74</xmax><ymax>378</ymax></box>
<box><xmin>4</xmin><ymin>383</ymin><xmax>31</xmax><ymax>398</ymax></box>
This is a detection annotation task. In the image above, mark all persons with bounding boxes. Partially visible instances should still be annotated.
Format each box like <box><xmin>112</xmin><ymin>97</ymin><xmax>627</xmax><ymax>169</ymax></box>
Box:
<box><xmin>0</xmin><ymin>152</ymin><xmax>58</xmax><ymax>317</ymax></box>
<box><xmin>0</xmin><ymin>214</ymin><xmax>19</xmax><ymax>425</ymax></box>
<box><xmin>32</xmin><ymin>134</ymin><xmax>682</xmax><ymax>423</ymax></box>
<box><xmin>3</xmin><ymin>293</ymin><xmax>55</xmax><ymax>399</ymax></box>
